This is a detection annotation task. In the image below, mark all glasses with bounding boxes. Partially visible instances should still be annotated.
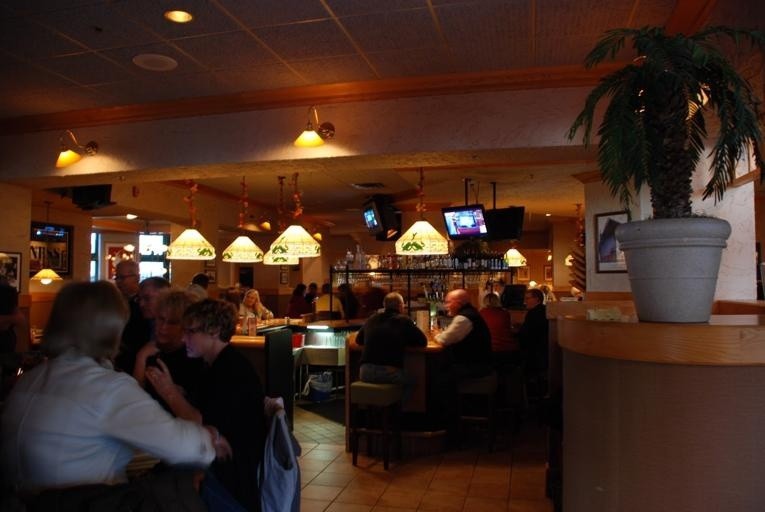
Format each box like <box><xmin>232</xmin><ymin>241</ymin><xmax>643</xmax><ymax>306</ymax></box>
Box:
<box><xmin>135</xmin><ymin>296</ymin><xmax>159</xmax><ymax>302</ymax></box>
<box><xmin>113</xmin><ymin>275</ymin><xmax>135</xmax><ymax>280</ymax></box>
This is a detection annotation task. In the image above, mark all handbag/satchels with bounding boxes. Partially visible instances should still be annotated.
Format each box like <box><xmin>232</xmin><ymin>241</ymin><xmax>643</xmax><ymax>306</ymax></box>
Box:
<box><xmin>260</xmin><ymin>409</ymin><xmax>299</xmax><ymax>512</ymax></box>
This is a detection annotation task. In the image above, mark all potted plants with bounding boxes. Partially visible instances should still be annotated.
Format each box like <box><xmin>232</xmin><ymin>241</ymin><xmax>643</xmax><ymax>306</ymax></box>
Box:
<box><xmin>564</xmin><ymin>21</ymin><xmax>765</xmax><ymax>324</ymax></box>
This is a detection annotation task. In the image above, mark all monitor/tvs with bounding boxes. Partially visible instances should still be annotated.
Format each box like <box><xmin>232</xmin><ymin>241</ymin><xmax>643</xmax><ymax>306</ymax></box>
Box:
<box><xmin>488</xmin><ymin>206</ymin><xmax>524</xmax><ymax>239</ymax></box>
<box><xmin>360</xmin><ymin>201</ymin><xmax>397</xmax><ymax>233</ymax></box>
<box><xmin>441</xmin><ymin>204</ymin><xmax>491</xmax><ymax>240</ymax></box>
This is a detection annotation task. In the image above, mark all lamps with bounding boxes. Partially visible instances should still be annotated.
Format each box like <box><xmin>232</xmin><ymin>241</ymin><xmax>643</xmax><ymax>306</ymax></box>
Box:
<box><xmin>164</xmin><ymin>1</ymin><xmax>195</xmax><ymax>23</ymax></box>
<box><xmin>31</xmin><ymin>201</ymin><xmax>65</xmax><ymax>286</ymax></box>
<box><xmin>262</xmin><ymin>175</ymin><xmax>299</xmax><ymax>266</ymax></box>
<box><xmin>222</xmin><ymin>177</ymin><xmax>262</xmax><ymax>263</ymax></box>
<box><xmin>270</xmin><ymin>173</ymin><xmax>320</xmax><ymax>259</ymax></box>
<box><xmin>164</xmin><ymin>180</ymin><xmax>216</xmax><ymax>261</ymax></box>
<box><xmin>504</xmin><ymin>239</ymin><xmax>527</xmax><ymax>268</ymax></box>
<box><xmin>290</xmin><ymin>103</ymin><xmax>336</xmax><ymax>146</ymax></box>
<box><xmin>394</xmin><ymin>168</ymin><xmax>449</xmax><ymax>256</ymax></box>
<box><xmin>103</xmin><ymin>244</ymin><xmax>136</xmax><ymax>264</ymax></box>
<box><xmin>54</xmin><ymin>129</ymin><xmax>99</xmax><ymax>170</ymax></box>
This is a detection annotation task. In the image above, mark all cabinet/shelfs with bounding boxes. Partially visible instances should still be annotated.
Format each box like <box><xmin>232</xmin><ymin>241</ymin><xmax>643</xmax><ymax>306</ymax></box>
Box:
<box><xmin>330</xmin><ymin>263</ymin><xmax>513</xmax><ymax>318</ymax></box>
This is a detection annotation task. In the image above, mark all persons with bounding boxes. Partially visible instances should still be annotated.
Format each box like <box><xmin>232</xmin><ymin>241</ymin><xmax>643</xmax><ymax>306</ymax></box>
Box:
<box><xmin>354</xmin><ymin>280</ymin><xmax>548</xmax><ymax>430</ymax></box>
<box><xmin>290</xmin><ymin>280</ymin><xmax>357</xmax><ymax>320</ymax></box>
<box><xmin>1</xmin><ymin>261</ymin><xmax>285</xmax><ymax>512</ymax></box>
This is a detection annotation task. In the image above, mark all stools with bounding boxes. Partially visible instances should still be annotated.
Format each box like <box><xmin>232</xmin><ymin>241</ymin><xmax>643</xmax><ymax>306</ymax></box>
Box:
<box><xmin>350</xmin><ymin>380</ymin><xmax>399</xmax><ymax>472</ymax></box>
<box><xmin>302</xmin><ymin>346</ymin><xmax>345</xmax><ymax>400</ymax></box>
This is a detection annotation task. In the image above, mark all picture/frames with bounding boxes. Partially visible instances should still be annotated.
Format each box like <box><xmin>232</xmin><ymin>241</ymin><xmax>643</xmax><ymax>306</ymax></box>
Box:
<box><xmin>105</xmin><ymin>243</ymin><xmax>137</xmax><ymax>283</ymax></box>
<box><xmin>593</xmin><ymin>211</ymin><xmax>630</xmax><ymax>274</ymax></box>
<box><xmin>0</xmin><ymin>251</ymin><xmax>21</xmax><ymax>294</ymax></box>
<box><xmin>518</xmin><ymin>263</ymin><xmax>531</xmax><ymax>282</ymax></box>
<box><xmin>543</xmin><ymin>264</ymin><xmax>553</xmax><ymax>282</ymax></box>
<box><xmin>279</xmin><ymin>262</ymin><xmax>290</xmax><ymax>285</ymax></box>
<box><xmin>28</xmin><ymin>220</ymin><xmax>72</xmax><ymax>276</ymax></box>
<box><xmin>203</xmin><ymin>253</ymin><xmax>217</xmax><ymax>284</ymax></box>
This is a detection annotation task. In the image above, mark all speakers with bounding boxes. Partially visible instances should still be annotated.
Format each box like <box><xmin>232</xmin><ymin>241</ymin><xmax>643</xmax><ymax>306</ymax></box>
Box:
<box><xmin>73</xmin><ymin>184</ymin><xmax>112</xmax><ymax>209</ymax></box>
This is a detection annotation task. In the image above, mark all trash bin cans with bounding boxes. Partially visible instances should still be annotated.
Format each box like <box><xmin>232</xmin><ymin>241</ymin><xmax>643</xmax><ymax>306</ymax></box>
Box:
<box><xmin>309</xmin><ymin>375</ymin><xmax>332</xmax><ymax>413</ymax></box>
